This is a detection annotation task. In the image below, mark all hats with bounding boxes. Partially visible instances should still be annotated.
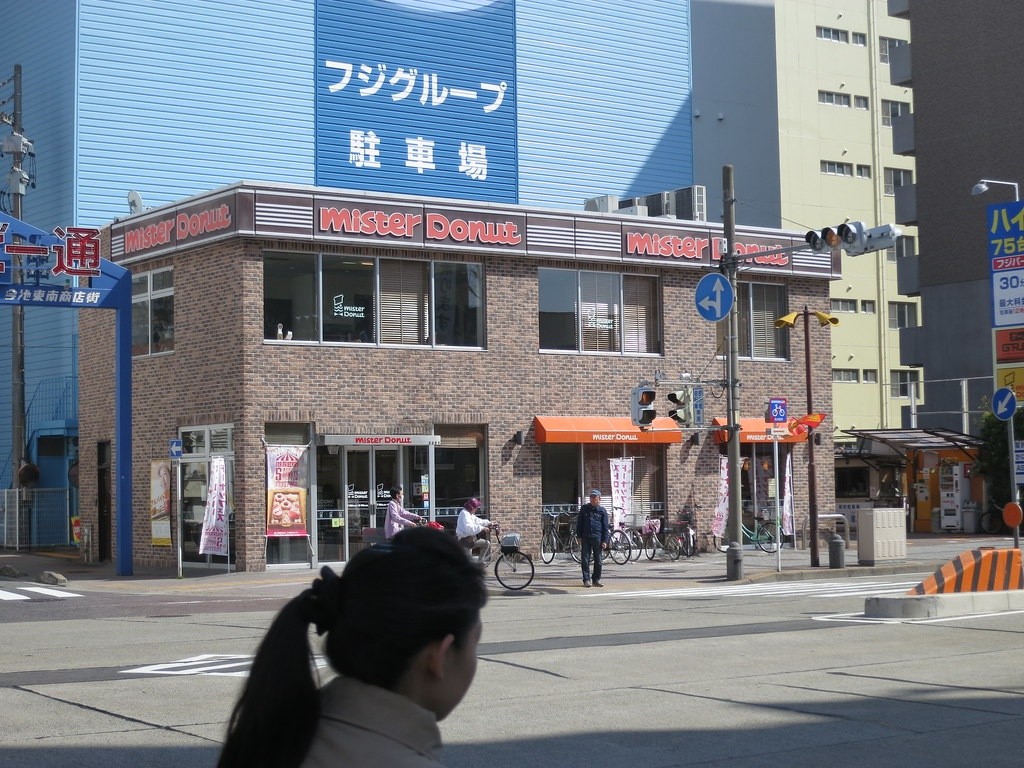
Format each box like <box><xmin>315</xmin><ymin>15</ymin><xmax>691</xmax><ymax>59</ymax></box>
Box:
<box><xmin>589</xmin><ymin>490</ymin><xmax>601</xmax><ymax>497</ymax></box>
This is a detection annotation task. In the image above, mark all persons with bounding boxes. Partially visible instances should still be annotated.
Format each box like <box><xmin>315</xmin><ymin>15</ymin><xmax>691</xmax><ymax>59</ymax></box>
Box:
<box><xmin>576</xmin><ymin>489</ymin><xmax>609</xmax><ymax>587</ymax></box>
<box><xmin>217</xmin><ymin>527</ymin><xmax>487</xmax><ymax>768</ymax></box>
<box><xmin>385</xmin><ymin>485</ymin><xmax>423</xmax><ymax>537</ymax></box>
<box><xmin>149</xmin><ymin>465</ymin><xmax>170</xmax><ymax>518</ymax></box>
<box><xmin>454</xmin><ymin>498</ymin><xmax>498</xmax><ymax>565</ymax></box>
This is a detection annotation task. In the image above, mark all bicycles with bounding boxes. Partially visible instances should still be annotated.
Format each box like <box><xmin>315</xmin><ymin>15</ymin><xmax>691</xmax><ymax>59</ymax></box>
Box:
<box><xmin>416</xmin><ymin>517</ymin><xmax>444</xmax><ymax>531</ymax></box>
<box><xmin>478</xmin><ymin>524</ymin><xmax>535</xmax><ymax>590</ymax></box>
<box><xmin>540</xmin><ymin>506</ymin><xmax>703</xmax><ymax>565</ymax></box>
<box><xmin>980</xmin><ymin>500</ymin><xmax>1004</xmax><ymax>535</ymax></box>
<box><xmin>713</xmin><ymin>507</ymin><xmax>785</xmax><ymax>554</ymax></box>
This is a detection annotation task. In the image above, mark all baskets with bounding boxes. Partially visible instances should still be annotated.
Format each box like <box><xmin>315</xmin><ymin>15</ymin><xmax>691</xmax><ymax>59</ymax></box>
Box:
<box><xmin>500</xmin><ymin>537</ymin><xmax>518</xmax><ymax>553</ymax></box>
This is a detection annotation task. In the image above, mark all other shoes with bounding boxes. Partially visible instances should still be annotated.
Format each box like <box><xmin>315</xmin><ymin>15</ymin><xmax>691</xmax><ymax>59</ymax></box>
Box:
<box><xmin>585</xmin><ymin>581</ymin><xmax>591</xmax><ymax>587</ymax></box>
<box><xmin>592</xmin><ymin>581</ymin><xmax>604</xmax><ymax>587</ymax></box>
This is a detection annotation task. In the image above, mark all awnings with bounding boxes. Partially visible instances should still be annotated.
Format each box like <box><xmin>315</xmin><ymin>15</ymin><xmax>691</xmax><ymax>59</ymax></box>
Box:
<box><xmin>713</xmin><ymin>417</ymin><xmax>808</xmax><ymax>444</ymax></box>
<box><xmin>534</xmin><ymin>416</ymin><xmax>684</xmax><ymax>445</ymax></box>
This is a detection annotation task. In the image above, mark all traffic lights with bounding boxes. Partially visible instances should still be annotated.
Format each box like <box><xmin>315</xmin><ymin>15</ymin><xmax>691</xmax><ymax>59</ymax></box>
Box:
<box><xmin>630</xmin><ymin>387</ymin><xmax>657</xmax><ymax>426</ymax></box>
<box><xmin>804</xmin><ymin>220</ymin><xmax>866</xmax><ymax>254</ymax></box>
<box><xmin>847</xmin><ymin>225</ymin><xmax>903</xmax><ymax>257</ymax></box>
<box><xmin>668</xmin><ymin>389</ymin><xmax>694</xmax><ymax>425</ymax></box>
